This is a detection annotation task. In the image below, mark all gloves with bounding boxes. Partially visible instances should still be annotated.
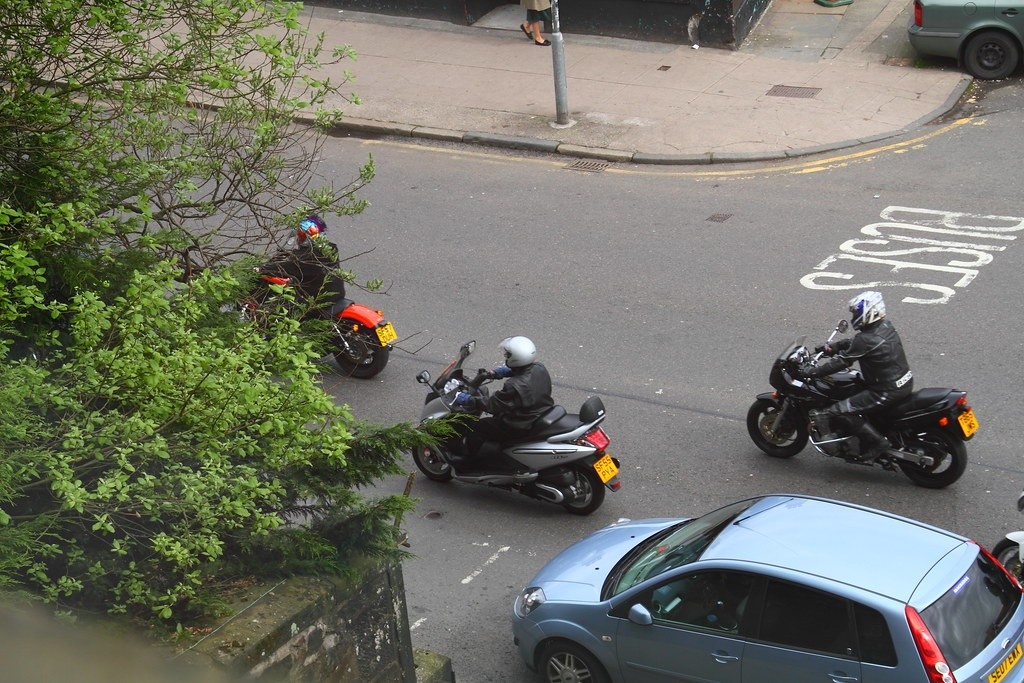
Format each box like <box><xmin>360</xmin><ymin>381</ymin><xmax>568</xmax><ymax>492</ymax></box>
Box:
<box><xmin>798</xmin><ymin>363</ymin><xmax>816</xmax><ymax>376</ymax></box>
<box><xmin>826</xmin><ymin>342</ymin><xmax>839</xmax><ymax>357</ymax></box>
<box><xmin>456</xmin><ymin>393</ymin><xmax>471</xmax><ymax>404</ymax></box>
<box><xmin>494</xmin><ymin>366</ymin><xmax>511</xmax><ymax>378</ymax></box>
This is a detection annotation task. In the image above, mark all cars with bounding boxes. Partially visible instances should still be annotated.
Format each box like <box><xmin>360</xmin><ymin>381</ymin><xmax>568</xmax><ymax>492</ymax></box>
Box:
<box><xmin>511</xmin><ymin>494</ymin><xmax>1024</xmax><ymax>683</ymax></box>
<box><xmin>908</xmin><ymin>1</ymin><xmax>1024</xmax><ymax>79</ymax></box>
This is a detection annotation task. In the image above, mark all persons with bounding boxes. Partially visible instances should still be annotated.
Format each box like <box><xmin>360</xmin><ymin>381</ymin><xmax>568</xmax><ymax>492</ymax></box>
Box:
<box><xmin>258</xmin><ymin>210</ymin><xmax>345</xmax><ymax>369</ymax></box>
<box><xmin>428</xmin><ymin>336</ymin><xmax>554</xmax><ymax>464</ymax></box>
<box><xmin>793</xmin><ymin>291</ymin><xmax>914</xmax><ymax>460</ymax></box>
<box><xmin>520</xmin><ymin>0</ymin><xmax>552</xmax><ymax>45</ymax></box>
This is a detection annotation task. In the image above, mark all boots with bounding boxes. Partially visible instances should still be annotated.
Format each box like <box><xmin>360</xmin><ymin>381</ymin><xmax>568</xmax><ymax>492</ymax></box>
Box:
<box><xmin>860</xmin><ymin>423</ymin><xmax>892</xmax><ymax>453</ymax></box>
<box><xmin>465</xmin><ymin>432</ymin><xmax>481</xmax><ymax>464</ymax></box>
<box><xmin>858</xmin><ymin>424</ymin><xmax>860</xmax><ymax>425</ymax></box>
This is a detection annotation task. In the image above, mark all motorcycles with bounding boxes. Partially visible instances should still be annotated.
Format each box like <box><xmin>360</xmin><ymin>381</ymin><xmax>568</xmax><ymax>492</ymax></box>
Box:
<box><xmin>994</xmin><ymin>497</ymin><xmax>1024</xmax><ymax>585</ymax></box>
<box><xmin>411</xmin><ymin>340</ymin><xmax>622</xmax><ymax>516</ymax></box>
<box><xmin>746</xmin><ymin>319</ymin><xmax>980</xmax><ymax>489</ymax></box>
<box><xmin>231</xmin><ymin>248</ymin><xmax>397</xmax><ymax>380</ymax></box>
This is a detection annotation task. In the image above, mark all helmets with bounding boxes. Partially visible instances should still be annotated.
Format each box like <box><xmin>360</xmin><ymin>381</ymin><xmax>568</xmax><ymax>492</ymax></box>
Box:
<box><xmin>297</xmin><ymin>220</ymin><xmax>319</xmax><ymax>245</ymax></box>
<box><xmin>497</xmin><ymin>336</ymin><xmax>537</xmax><ymax>367</ymax></box>
<box><xmin>847</xmin><ymin>291</ymin><xmax>886</xmax><ymax>330</ymax></box>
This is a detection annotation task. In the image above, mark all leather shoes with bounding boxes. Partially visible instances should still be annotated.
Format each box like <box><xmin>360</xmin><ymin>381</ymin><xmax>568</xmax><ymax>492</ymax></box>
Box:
<box><xmin>520</xmin><ymin>24</ymin><xmax>533</xmax><ymax>41</ymax></box>
<box><xmin>535</xmin><ymin>39</ymin><xmax>552</xmax><ymax>46</ymax></box>
